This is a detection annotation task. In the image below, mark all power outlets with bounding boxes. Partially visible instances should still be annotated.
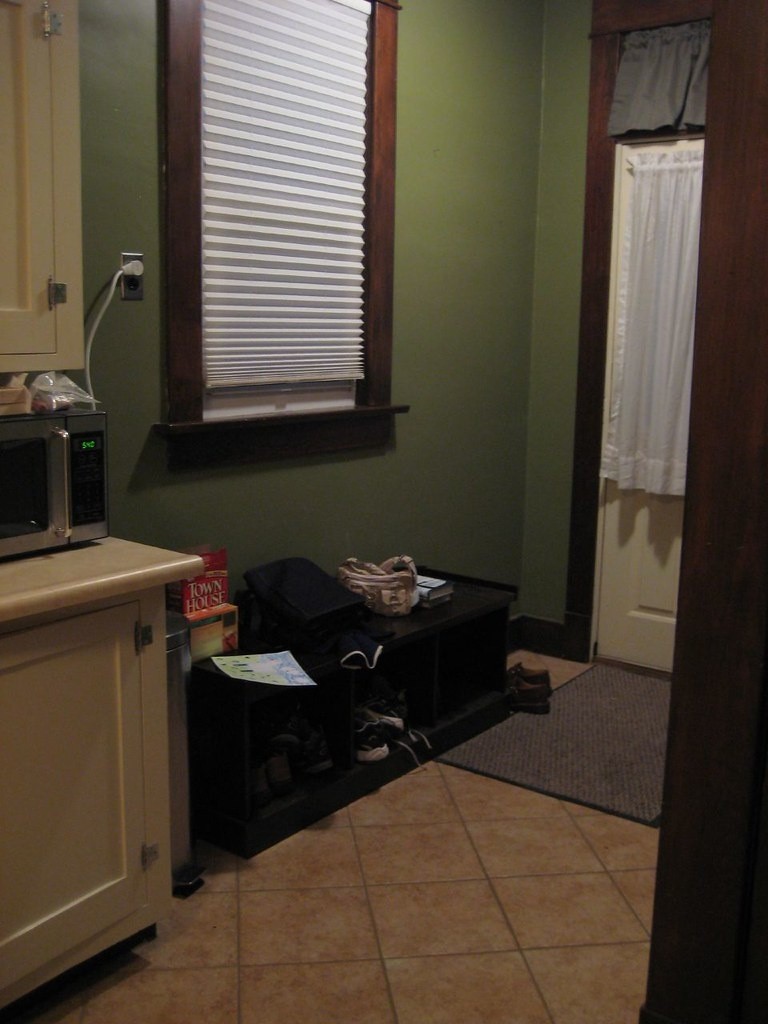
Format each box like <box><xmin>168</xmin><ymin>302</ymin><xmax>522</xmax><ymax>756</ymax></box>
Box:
<box><xmin>121</xmin><ymin>253</ymin><xmax>144</xmax><ymax>300</ymax></box>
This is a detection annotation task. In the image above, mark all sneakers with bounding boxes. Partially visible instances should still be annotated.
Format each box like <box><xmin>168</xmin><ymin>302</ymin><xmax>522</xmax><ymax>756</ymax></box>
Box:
<box><xmin>356</xmin><ymin>675</ymin><xmax>410</xmax><ymax>733</ymax></box>
<box><xmin>353</xmin><ymin>710</ymin><xmax>392</xmax><ymax>763</ymax></box>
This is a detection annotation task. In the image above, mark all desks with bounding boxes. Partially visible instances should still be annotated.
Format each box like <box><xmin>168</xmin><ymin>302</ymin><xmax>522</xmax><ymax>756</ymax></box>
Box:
<box><xmin>185</xmin><ymin>569</ymin><xmax>520</xmax><ymax>860</ymax></box>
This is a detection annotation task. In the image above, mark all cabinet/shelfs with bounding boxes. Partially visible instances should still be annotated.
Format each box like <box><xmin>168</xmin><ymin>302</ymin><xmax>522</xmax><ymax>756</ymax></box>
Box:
<box><xmin>0</xmin><ymin>0</ymin><xmax>85</xmax><ymax>373</ymax></box>
<box><xmin>0</xmin><ymin>535</ymin><xmax>204</xmax><ymax>1011</ymax></box>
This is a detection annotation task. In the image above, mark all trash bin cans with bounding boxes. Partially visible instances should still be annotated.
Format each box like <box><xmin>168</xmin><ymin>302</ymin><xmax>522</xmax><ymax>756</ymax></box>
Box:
<box><xmin>162</xmin><ymin>609</ymin><xmax>192</xmax><ymax>871</ymax></box>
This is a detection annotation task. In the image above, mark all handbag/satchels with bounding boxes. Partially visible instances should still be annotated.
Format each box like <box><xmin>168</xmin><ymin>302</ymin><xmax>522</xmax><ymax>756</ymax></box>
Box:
<box><xmin>334</xmin><ymin>553</ymin><xmax>418</xmax><ymax>617</ymax></box>
<box><xmin>241</xmin><ymin>556</ymin><xmax>367</xmax><ymax>638</ymax></box>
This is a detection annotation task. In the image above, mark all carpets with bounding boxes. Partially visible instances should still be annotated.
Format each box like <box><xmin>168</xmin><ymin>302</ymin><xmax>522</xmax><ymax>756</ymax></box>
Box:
<box><xmin>434</xmin><ymin>658</ymin><xmax>672</xmax><ymax>829</ymax></box>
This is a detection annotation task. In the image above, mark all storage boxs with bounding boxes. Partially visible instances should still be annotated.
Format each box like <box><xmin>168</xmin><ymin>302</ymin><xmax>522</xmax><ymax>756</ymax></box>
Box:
<box><xmin>183</xmin><ymin>603</ymin><xmax>237</xmax><ymax>662</ymax></box>
<box><xmin>166</xmin><ymin>544</ymin><xmax>227</xmax><ymax>613</ymax></box>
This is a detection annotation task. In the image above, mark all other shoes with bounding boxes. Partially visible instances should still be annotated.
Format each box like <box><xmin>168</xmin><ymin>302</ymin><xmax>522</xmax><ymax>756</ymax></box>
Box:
<box><xmin>507</xmin><ymin>661</ymin><xmax>551</xmax><ymax>687</ymax></box>
<box><xmin>507</xmin><ymin>674</ymin><xmax>550</xmax><ymax>714</ymax></box>
<box><xmin>272</xmin><ymin>713</ymin><xmax>335</xmax><ymax>774</ymax></box>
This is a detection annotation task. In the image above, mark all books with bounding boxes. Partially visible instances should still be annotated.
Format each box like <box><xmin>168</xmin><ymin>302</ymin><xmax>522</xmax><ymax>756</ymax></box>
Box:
<box><xmin>416</xmin><ymin>574</ymin><xmax>454</xmax><ymax>608</ymax></box>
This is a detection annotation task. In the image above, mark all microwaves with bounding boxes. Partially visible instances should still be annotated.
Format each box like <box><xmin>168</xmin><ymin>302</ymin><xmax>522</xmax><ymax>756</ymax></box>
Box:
<box><xmin>0</xmin><ymin>407</ymin><xmax>113</xmax><ymax>560</ymax></box>
